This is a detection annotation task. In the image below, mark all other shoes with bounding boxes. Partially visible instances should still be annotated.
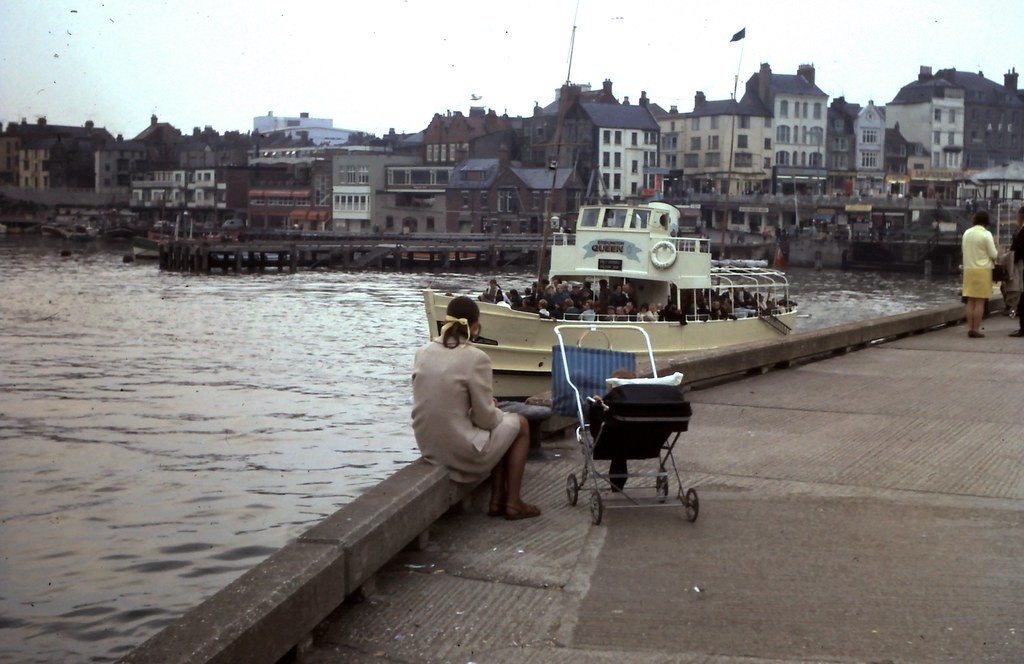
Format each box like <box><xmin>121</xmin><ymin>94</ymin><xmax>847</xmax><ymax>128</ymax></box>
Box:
<box><xmin>1009</xmin><ymin>330</ymin><xmax>1024</xmax><ymax>337</ymax></box>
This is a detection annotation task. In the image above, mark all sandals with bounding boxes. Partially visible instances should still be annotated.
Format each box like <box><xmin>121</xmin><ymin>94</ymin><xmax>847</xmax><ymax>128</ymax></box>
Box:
<box><xmin>488</xmin><ymin>499</ymin><xmax>541</xmax><ymax>520</ymax></box>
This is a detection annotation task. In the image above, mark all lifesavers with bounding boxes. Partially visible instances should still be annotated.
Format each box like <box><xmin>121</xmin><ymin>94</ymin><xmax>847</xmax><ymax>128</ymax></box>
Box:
<box><xmin>648</xmin><ymin>241</ymin><xmax>677</xmax><ymax>269</ymax></box>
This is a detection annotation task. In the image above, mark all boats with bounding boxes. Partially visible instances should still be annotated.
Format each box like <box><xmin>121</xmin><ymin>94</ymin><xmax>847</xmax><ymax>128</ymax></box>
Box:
<box><xmin>62</xmin><ymin>226</ymin><xmax>99</xmax><ymax>241</ymax></box>
<box><xmin>422</xmin><ymin>28</ymin><xmax>798</xmax><ymax>401</ymax></box>
<box><xmin>41</xmin><ymin>221</ymin><xmax>65</xmax><ymax>236</ymax></box>
<box><xmin>131</xmin><ymin>231</ymin><xmax>240</xmax><ymax>259</ymax></box>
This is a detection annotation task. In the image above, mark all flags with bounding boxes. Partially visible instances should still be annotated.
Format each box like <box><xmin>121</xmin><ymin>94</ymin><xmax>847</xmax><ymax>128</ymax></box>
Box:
<box><xmin>730</xmin><ymin>27</ymin><xmax>745</xmax><ymax>42</ymax></box>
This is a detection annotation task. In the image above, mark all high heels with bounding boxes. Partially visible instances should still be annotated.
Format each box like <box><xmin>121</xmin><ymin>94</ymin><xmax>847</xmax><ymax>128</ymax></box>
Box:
<box><xmin>969</xmin><ymin>331</ymin><xmax>984</xmax><ymax>338</ymax></box>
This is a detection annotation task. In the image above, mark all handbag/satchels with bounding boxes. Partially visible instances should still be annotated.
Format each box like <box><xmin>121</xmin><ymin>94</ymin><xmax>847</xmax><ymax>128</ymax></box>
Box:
<box><xmin>992</xmin><ymin>264</ymin><xmax>1010</xmax><ymax>281</ymax></box>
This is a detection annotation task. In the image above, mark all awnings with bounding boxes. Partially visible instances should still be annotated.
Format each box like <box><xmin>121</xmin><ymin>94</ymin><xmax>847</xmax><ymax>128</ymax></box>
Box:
<box><xmin>290</xmin><ymin>210</ymin><xmax>328</xmax><ymax>220</ymax></box>
<box><xmin>249</xmin><ymin>187</ymin><xmax>312</xmax><ymax>198</ymax></box>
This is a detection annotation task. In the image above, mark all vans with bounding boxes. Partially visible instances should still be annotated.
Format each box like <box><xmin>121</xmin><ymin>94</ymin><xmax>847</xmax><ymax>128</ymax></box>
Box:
<box><xmin>222</xmin><ymin>219</ymin><xmax>244</xmax><ymax>230</ymax></box>
<box><xmin>153</xmin><ymin>220</ymin><xmax>171</xmax><ymax>227</ymax></box>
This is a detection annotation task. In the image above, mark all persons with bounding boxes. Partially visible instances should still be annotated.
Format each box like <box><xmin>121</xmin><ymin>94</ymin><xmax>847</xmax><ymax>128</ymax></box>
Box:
<box><xmin>763</xmin><ymin>228</ymin><xmax>768</xmax><ymax>241</ymax></box>
<box><xmin>1008</xmin><ymin>206</ymin><xmax>1024</xmax><ymax>338</ymax></box>
<box><xmin>478</xmin><ymin>277</ymin><xmax>787</xmax><ymax>322</ymax></box>
<box><xmin>607</xmin><ymin>369</ymin><xmax>636</xmax><ymax>393</ymax></box>
<box><xmin>412</xmin><ymin>296</ymin><xmax>542</xmax><ymax>520</ymax></box>
<box><xmin>962</xmin><ymin>211</ymin><xmax>1002</xmax><ymax>338</ymax></box>
<box><xmin>729</xmin><ymin>229</ymin><xmax>745</xmax><ymax>244</ymax></box>
<box><xmin>775</xmin><ymin>225</ymin><xmax>782</xmax><ymax>242</ymax></box>
